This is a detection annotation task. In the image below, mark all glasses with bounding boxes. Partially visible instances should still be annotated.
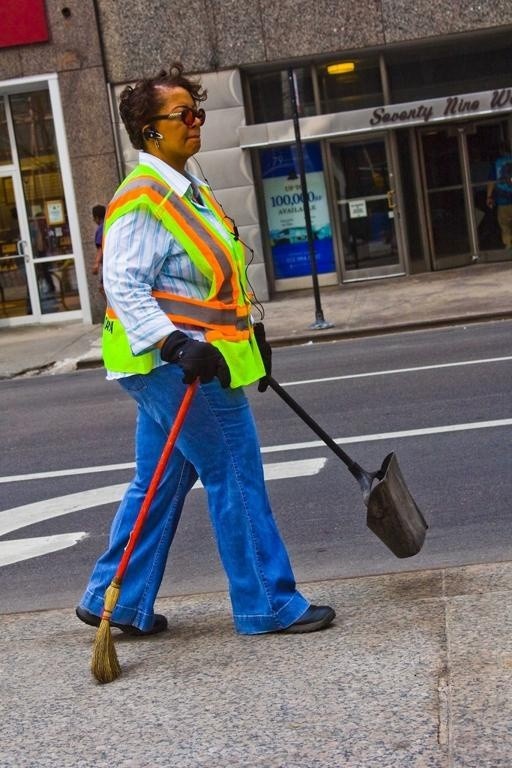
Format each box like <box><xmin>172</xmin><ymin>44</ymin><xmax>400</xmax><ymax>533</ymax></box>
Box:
<box><xmin>146</xmin><ymin>108</ymin><xmax>206</xmax><ymax>126</ymax></box>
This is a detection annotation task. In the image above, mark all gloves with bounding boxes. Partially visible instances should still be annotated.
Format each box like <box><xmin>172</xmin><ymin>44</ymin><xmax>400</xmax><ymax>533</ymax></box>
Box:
<box><xmin>253</xmin><ymin>322</ymin><xmax>272</xmax><ymax>392</ymax></box>
<box><xmin>160</xmin><ymin>330</ymin><xmax>231</xmax><ymax>388</ymax></box>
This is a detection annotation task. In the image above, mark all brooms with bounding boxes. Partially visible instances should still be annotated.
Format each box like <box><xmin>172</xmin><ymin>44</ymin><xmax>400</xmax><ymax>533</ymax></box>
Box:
<box><xmin>89</xmin><ymin>376</ymin><xmax>200</xmax><ymax>686</ymax></box>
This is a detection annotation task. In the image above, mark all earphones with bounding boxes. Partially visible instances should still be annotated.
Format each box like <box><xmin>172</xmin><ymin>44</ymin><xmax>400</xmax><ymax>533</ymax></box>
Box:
<box><xmin>144</xmin><ymin>131</ymin><xmax>162</xmax><ymax>139</ymax></box>
<box><xmin>234</xmin><ymin>226</ymin><xmax>239</xmax><ymax>241</ymax></box>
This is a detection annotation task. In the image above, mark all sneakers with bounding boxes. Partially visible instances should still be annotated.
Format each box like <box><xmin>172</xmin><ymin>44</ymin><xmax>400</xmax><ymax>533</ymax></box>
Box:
<box><xmin>271</xmin><ymin>605</ymin><xmax>336</xmax><ymax>634</ymax></box>
<box><xmin>76</xmin><ymin>603</ymin><xmax>168</xmax><ymax>637</ymax></box>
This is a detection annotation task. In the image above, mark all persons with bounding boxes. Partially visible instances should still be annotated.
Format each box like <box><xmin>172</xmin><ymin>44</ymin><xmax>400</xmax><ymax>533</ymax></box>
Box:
<box><xmin>74</xmin><ymin>61</ymin><xmax>337</xmax><ymax>634</ymax></box>
<box><xmin>10</xmin><ymin>207</ymin><xmax>55</xmax><ymax>314</ymax></box>
<box><xmin>486</xmin><ymin>141</ymin><xmax>512</xmax><ymax>250</ymax></box>
<box><xmin>91</xmin><ymin>205</ymin><xmax>107</xmax><ymax>302</ymax></box>
